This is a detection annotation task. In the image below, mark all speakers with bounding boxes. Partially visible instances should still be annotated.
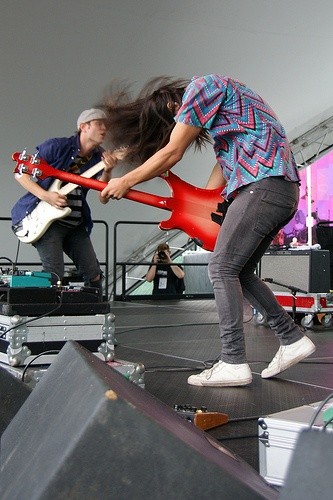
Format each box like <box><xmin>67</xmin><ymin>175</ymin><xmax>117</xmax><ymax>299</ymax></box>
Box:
<box><xmin>259</xmin><ymin>249</ymin><xmax>332</xmax><ymax>293</ymax></box>
<box><xmin>0</xmin><ymin>340</ymin><xmax>282</xmax><ymax>500</ymax></box>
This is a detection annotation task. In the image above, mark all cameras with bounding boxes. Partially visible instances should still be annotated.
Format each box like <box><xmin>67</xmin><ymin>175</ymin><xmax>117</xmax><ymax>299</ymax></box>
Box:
<box><xmin>157</xmin><ymin>251</ymin><xmax>166</xmax><ymax>259</ymax></box>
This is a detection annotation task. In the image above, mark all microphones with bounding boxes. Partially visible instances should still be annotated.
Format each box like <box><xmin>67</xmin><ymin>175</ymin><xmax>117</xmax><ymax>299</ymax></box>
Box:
<box><xmin>262</xmin><ymin>278</ymin><xmax>273</xmax><ymax>282</ymax></box>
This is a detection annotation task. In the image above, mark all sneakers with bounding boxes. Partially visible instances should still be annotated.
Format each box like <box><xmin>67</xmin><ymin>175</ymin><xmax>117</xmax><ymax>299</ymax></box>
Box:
<box><xmin>261</xmin><ymin>336</ymin><xmax>316</xmax><ymax>378</ymax></box>
<box><xmin>187</xmin><ymin>360</ymin><xmax>253</xmax><ymax>387</ymax></box>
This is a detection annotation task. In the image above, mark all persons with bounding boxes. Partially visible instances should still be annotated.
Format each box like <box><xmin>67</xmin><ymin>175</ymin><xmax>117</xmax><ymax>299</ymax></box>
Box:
<box><xmin>92</xmin><ymin>73</ymin><xmax>317</xmax><ymax>387</ymax></box>
<box><xmin>146</xmin><ymin>244</ymin><xmax>185</xmax><ymax>295</ymax></box>
<box><xmin>12</xmin><ymin>109</ymin><xmax>107</xmax><ymax>295</ymax></box>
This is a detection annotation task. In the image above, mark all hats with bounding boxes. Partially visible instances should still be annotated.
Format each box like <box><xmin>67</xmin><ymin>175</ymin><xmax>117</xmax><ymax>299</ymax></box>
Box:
<box><xmin>77</xmin><ymin>108</ymin><xmax>108</xmax><ymax>132</ymax></box>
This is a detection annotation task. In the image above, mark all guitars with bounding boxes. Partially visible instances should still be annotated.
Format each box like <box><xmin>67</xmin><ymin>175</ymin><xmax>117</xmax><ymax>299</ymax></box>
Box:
<box><xmin>13</xmin><ymin>145</ymin><xmax>140</xmax><ymax>245</ymax></box>
<box><xmin>9</xmin><ymin>145</ymin><xmax>238</xmax><ymax>254</ymax></box>
